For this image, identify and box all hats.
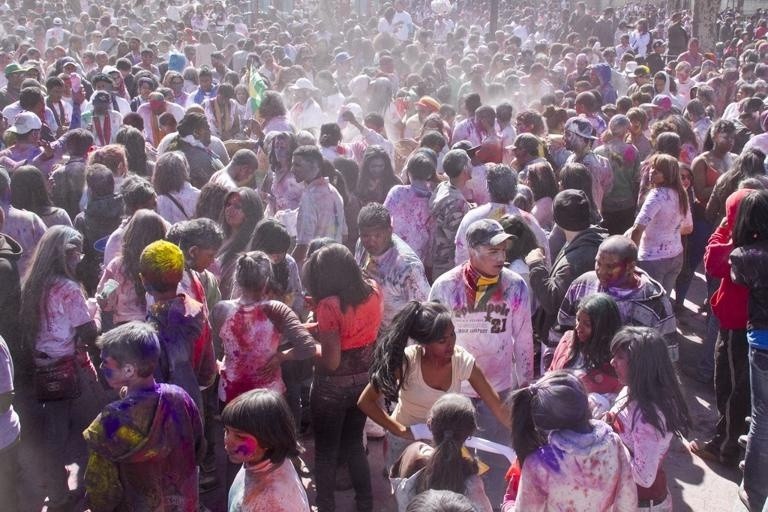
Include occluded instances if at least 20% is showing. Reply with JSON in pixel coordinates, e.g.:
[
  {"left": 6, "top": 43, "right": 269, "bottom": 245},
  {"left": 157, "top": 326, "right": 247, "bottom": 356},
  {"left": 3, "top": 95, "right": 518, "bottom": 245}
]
[
  {"left": 52, "top": 18, "right": 62, "bottom": 25},
  {"left": 336, "top": 52, "right": 353, "bottom": 64},
  {"left": 553, "top": 189, "right": 590, "bottom": 230},
  {"left": 505, "top": 134, "right": 542, "bottom": 150},
  {"left": 7, "top": 111, "right": 41, "bottom": 135},
  {"left": 628, "top": 65, "right": 650, "bottom": 79},
  {"left": 290, "top": 78, "right": 317, "bottom": 91},
  {"left": 466, "top": 219, "right": 517, "bottom": 246},
  {"left": 563, "top": 118, "right": 598, "bottom": 139},
  {"left": 414, "top": 96, "right": 439, "bottom": 113},
  {"left": 5, "top": 63, "right": 27, "bottom": 76},
  {"left": 451, "top": 140, "right": 481, "bottom": 152}
]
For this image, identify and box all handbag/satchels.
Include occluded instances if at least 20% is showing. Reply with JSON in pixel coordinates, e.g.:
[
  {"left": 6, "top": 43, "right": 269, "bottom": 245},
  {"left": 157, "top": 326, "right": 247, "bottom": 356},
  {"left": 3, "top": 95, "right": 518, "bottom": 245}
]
[{"left": 35, "top": 362, "right": 79, "bottom": 400}]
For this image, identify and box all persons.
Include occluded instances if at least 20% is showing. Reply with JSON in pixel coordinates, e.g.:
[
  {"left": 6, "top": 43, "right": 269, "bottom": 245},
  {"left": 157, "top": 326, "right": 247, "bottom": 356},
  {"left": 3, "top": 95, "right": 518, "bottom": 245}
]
[{"left": 0, "top": 0, "right": 768, "bottom": 512}]
